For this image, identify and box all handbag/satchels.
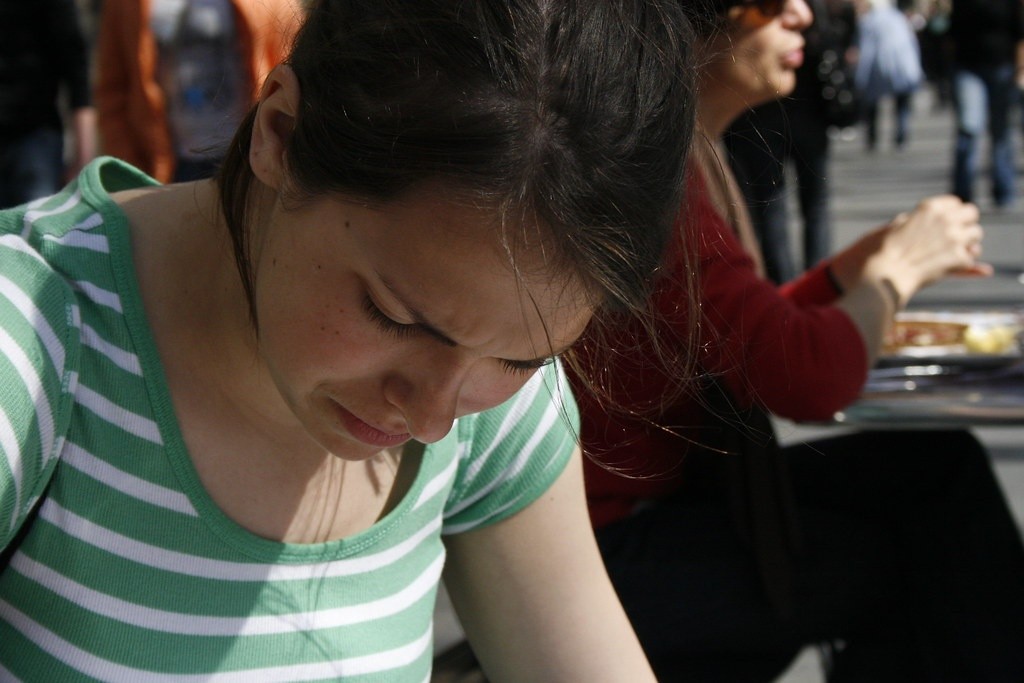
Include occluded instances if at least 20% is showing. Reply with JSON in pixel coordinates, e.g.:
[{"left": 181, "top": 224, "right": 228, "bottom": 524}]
[{"left": 817, "top": 54, "right": 859, "bottom": 128}]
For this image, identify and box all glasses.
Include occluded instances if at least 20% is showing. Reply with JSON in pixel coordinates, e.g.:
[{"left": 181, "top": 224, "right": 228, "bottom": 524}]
[{"left": 702, "top": 0, "right": 786, "bottom": 48}]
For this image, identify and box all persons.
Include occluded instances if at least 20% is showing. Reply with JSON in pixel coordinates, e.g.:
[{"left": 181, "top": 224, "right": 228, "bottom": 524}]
[{"left": 0, "top": 0, "right": 1024, "bottom": 683}]
